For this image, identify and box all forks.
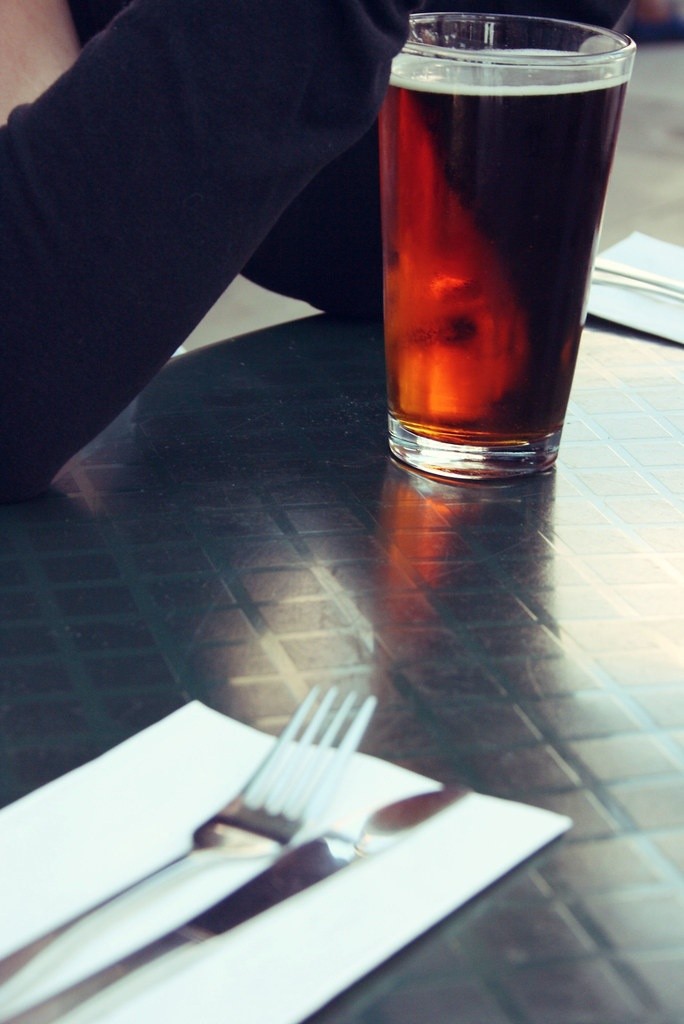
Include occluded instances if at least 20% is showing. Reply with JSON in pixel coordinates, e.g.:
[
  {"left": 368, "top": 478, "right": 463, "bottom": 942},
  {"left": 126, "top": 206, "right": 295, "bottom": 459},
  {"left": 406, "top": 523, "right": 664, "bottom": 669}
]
[{"left": 0, "top": 683, "right": 377, "bottom": 1022}]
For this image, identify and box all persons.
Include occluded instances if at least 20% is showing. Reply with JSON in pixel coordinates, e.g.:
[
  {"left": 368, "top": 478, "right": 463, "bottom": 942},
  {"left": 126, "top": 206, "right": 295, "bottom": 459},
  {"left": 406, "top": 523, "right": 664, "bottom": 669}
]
[{"left": 1, "top": 0, "right": 631, "bottom": 498}]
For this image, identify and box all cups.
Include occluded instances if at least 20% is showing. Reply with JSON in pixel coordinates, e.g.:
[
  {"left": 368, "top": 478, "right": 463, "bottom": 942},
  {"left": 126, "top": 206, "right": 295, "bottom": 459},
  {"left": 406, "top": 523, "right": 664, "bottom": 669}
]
[{"left": 375, "top": 10, "right": 636, "bottom": 490}]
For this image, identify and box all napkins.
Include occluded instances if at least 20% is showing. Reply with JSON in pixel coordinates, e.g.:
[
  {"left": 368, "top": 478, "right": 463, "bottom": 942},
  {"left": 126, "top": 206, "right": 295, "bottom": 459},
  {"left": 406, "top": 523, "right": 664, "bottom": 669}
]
[
  {"left": 588, "top": 231, "right": 684, "bottom": 348},
  {"left": 0, "top": 700, "right": 572, "bottom": 1024}
]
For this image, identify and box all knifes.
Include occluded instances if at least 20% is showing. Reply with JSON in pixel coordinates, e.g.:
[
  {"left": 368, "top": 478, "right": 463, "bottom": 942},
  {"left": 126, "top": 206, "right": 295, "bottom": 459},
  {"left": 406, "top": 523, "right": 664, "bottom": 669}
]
[{"left": 15, "top": 785, "right": 471, "bottom": 1023}]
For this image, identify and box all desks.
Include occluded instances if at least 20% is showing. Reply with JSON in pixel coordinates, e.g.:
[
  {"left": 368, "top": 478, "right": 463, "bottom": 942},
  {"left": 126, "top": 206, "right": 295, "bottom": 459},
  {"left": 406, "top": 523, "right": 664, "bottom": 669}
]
[{"left": 0, "top": 292, "right": 684, "bottom": 1023}]
